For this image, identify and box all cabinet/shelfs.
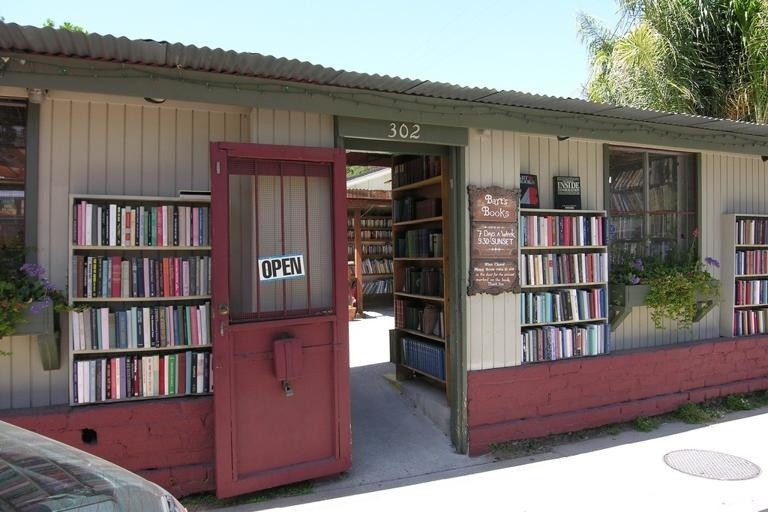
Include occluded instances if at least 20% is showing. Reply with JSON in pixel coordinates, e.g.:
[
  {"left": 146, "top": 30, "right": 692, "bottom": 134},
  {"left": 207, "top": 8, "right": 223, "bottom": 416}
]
[
  {"left": 718, "top": 210, "right": 767, "bottom": 340},
  {"left": 612, "top": 155, "right": 698, "bottom": 271},
  {"left": 345, "top": 197, "right": 393, "bottom": 312},
  {"left": 385, "top": 152, "right": 451, "bottom": 403},
  {"left": 63, "top": 190, "right": 222, "bottom": 408},
  {"left": 517, "top": 204, "right": 614, "bottom": 365}
]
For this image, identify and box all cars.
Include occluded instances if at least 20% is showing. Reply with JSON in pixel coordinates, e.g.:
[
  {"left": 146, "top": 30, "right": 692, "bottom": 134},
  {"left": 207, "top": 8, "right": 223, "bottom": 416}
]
[{"left": 0, "top": 419, "right": 190, "bottom": 510}]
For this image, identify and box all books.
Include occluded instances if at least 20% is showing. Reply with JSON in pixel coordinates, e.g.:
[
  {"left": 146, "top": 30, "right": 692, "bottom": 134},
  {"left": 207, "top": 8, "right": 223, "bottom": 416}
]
[
  {"left": 394, "top": 153, "right": 445, "bottom": 380},
  {"left": 72, "top": 200, "right": 213, "bottom": 405},
  {"left": 734, "top": 218, "right": 768, "bottom": 337},
  {"left": 345, "top": 214, "right": 393, "bottom": 296},
  {"left": 610, "top": 154, "right": 677, "bottom": 272},
  {"left": 518, "top": 215, "right": 608, "bottom": 363}
]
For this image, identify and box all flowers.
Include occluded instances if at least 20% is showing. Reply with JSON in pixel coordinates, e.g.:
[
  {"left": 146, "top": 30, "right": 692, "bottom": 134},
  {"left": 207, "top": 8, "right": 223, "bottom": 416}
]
[
  {"left": 0, "top": 254, "right": 94, "bottom": 358},
  {"left": 614, "top": 226, "right": 722, "bottom": 290}
]
